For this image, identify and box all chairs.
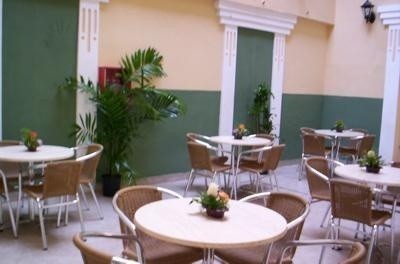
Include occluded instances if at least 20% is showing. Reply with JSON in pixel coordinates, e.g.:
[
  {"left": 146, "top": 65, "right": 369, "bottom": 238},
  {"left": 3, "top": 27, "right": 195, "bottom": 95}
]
[
  {"left": 296, "top": 125, "right": 376, "bottom": 179},
  {"left": 210, "top": 192, "right": 310, "bottom": 264},
  {"left": 304, "top": 156, "right": 345, "bottom": 227},
  {"left": 375, "top": 163, "right": 400, "bottom": 219},
  {"left": 0, "top": 140, "right": 104, "bottom": 249},
  {"left": 328, "top": 178, "right": 392, "bottom": 264},
  {"left": 112, "top": 185, "right": 204, "bottom": 264},
  {"left": 278, "top": 239, "right": 367, "bottom": 264},
  {"left": 187, "top": 132, "right": 286, "bottom": 196},
  {"left": 73, "top": 231, "right": 146, "bottom": 264}
]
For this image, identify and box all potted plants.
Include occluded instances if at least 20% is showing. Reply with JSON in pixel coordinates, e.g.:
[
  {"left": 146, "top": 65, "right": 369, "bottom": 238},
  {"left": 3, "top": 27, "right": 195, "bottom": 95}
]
[
  {"left": 54, "top": 47, "right": 186, "bottom": 197},
  {"left": 245, "top": 80, "right": 281, "bottom": 175},
  {"left": 334, "top": 121, "right": 344, "bottom": 132}
]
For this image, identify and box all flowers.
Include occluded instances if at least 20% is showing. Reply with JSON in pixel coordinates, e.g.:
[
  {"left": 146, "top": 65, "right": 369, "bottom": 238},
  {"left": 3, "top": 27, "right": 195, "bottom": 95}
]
[
  {"left": 232, "top": 124, "right": 247, "bottom": 139},
  {"left": 20, "top": 128, "right": 40, "bottom": 149},
  {"left": 190, "top": 181, "right": 230, "bottom": 208},
  {"left": 358, "top": 149, "right": 382, "bottom": 167}
]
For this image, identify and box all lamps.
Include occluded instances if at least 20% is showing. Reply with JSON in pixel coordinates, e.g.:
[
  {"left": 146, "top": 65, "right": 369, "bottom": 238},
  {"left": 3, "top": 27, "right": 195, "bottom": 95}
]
[{"left": 360, "top": 0, "right": 378, "bottom": 25}]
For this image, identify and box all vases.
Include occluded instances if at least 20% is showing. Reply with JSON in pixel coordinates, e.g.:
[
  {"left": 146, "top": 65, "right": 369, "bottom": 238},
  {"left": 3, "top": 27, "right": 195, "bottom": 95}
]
[
  {"left": 207, "top": 207, "right": 223, "bottom": 218},
  {"left": 365, "top": 164, "right": 380, "bottom": 174}
]
